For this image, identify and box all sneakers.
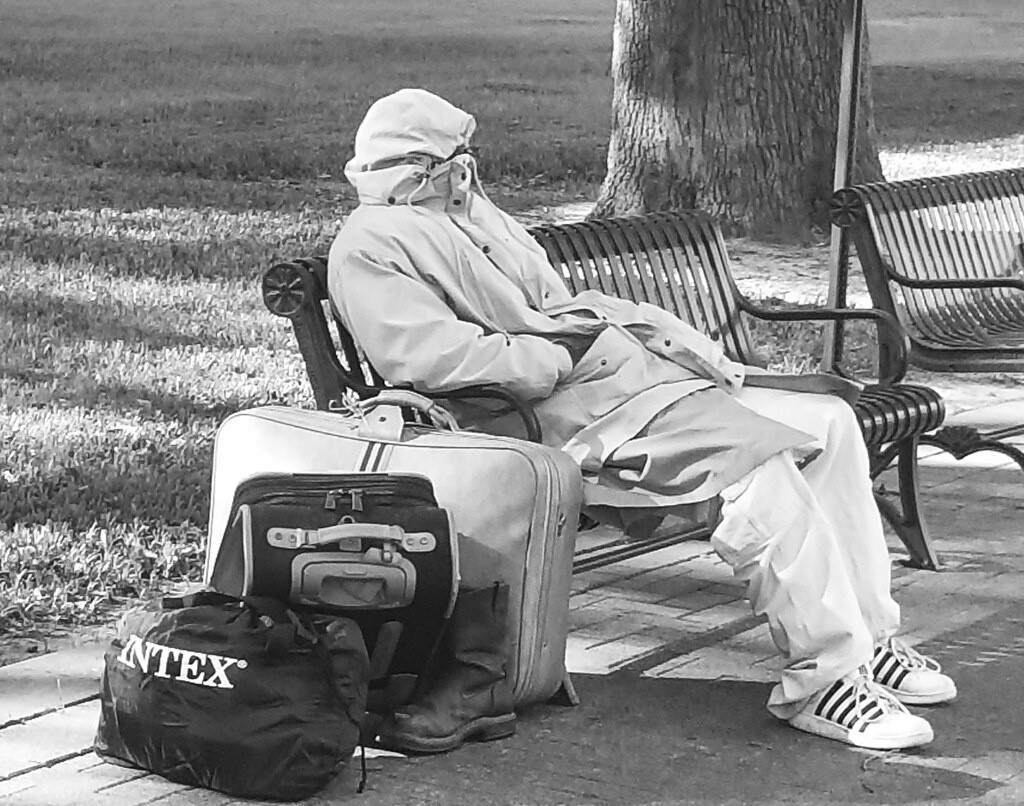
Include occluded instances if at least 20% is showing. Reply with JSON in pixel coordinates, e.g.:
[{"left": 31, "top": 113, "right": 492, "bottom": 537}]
[
  {"left": 869, "top": 636, "right": 958, "bottom": 703},
  {"left": 787, "top": 661, "right": 935, "bottom": 750}
]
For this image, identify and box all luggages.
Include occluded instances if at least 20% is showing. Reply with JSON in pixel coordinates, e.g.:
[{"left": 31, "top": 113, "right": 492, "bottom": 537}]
[{"left": 205, "top": 472, "right": 460, "bottom": 733}]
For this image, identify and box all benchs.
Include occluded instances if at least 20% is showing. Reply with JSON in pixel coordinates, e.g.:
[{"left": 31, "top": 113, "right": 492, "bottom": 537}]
[
  {"left": 262, "top": 209, "right": 945, "bottom": 706},
  {"left": 830, "top": 166, "right": 1024, "bottom": 473}
]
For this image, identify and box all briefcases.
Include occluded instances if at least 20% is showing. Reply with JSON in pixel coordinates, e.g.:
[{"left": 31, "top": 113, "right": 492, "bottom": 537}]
[{"left": 202, "top": 387, "right": 584, "bottom": 707}]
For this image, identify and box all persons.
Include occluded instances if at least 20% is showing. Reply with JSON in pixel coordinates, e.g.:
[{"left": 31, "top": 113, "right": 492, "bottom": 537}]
[{"left": 329, "top": 87, "right": 957, "bottom": 749}]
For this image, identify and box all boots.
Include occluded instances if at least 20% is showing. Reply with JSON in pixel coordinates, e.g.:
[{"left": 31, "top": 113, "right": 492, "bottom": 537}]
[{"left": 376, "top": 580, "right": 516, "bottom": 753}]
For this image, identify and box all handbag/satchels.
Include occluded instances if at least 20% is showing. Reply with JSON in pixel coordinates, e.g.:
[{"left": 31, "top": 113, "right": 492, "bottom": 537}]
[{"left": 91, "top": 587, "right": 370, "bottom": 802}]
